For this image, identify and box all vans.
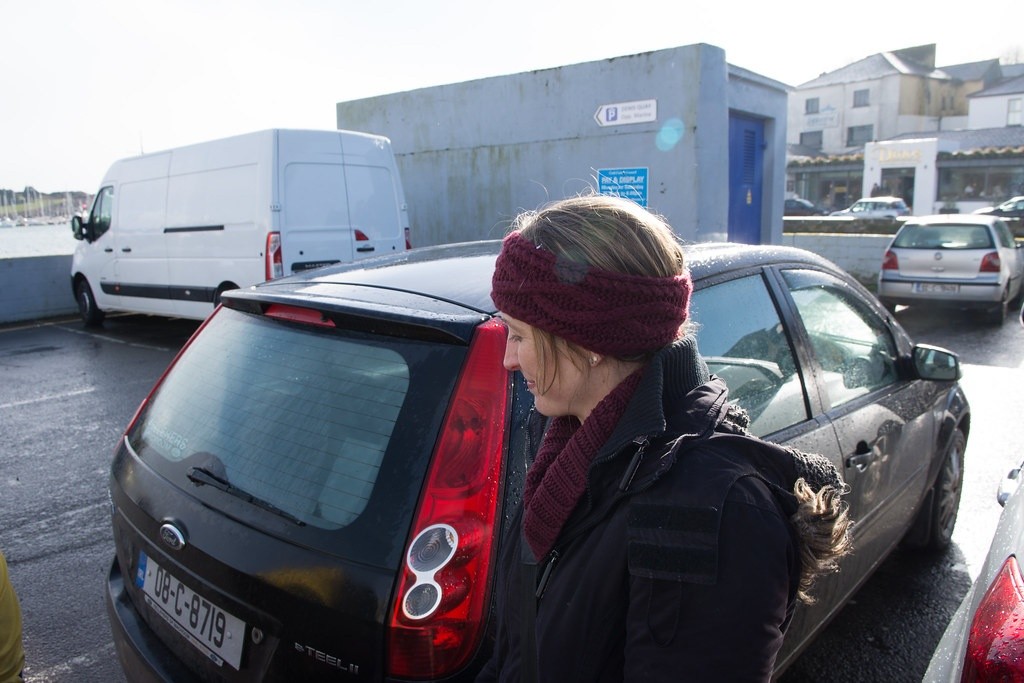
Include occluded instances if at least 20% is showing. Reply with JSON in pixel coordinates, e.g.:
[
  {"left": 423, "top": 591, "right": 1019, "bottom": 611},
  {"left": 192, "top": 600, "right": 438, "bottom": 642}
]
[{"left": 68, "top": 126, "right": 410, "bottom": 332}]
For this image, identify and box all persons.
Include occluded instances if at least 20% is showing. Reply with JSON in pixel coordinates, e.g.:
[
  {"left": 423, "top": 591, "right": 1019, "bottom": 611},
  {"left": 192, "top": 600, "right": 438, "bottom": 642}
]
[{"left": 491, "top": 192, "right": 854, "bottom": 683}]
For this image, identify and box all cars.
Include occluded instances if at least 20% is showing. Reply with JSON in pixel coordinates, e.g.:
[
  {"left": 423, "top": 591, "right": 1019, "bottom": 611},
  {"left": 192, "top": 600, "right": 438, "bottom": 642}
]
[
  {"left": 877, "top": 214, "right": 1023, "bottom": 327},
  {"left": 828, "top": 196, "right": 911, "bottom": 222},
  {"left": 784, "top": 198, "right": 830, "bottom": 217},
  {"left": 971, "top": 196, "right": 1024, "bottom": 218},
  {"left": 102, "top": 235, "right": 976, "bottom": 683}
]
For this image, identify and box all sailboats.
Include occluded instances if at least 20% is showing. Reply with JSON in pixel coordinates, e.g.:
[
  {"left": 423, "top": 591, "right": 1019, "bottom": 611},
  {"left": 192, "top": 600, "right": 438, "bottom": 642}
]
[{"left": 0, "top": 183, "right": 98, "bottom": 227}]
